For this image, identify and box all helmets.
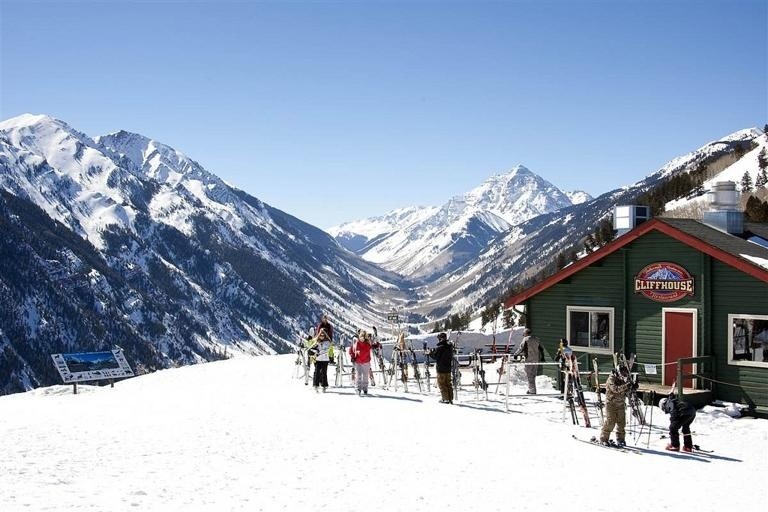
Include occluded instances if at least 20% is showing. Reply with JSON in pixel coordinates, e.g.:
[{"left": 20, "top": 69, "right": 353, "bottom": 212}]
[{"left": 658, "top": 398, "right": 668, "bottom": 411}]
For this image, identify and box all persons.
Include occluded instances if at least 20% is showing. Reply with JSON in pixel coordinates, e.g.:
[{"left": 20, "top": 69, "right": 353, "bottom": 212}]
[
  {"left": 303, "top": 314, "right": 334, "bottom": 393},
  {"left": 348, "top": 328, "right": 383, "bottom": 395},
  {"left": 513, "top": 328, "right": 545, "bottom": 395},
  {"left": 425, "top": 333, "right": 455, "bottom": 404},
  {"left": 658, "top": 397, "right": 696, "bottom": 453},
  {"left": 600, "top": 364, "right": 639, "bottom": 448},
  {"left": 553, "top": 338, "right": 576, "bottom": 400}
]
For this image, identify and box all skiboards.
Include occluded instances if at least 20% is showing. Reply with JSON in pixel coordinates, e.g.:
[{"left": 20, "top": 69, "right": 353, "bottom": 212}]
[
  {"left": 649, "top": 441, "right": 714, "bottom": 459},
  {"left": 369, "top": 325, "right": 516, "bottom": 401},
  {"left": 561, "top": 352, "right": 645, "bottom": 428},
  {"left": 334, "top": 330, "right": 361, "bottom": 386},
  {"left": 572, "top": 435, "right": 643, "bottom": 456}
]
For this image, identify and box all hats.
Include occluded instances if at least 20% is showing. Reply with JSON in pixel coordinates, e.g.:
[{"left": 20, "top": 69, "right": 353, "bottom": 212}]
[
  {"left": 522, "top": 328, "right": 531, "bottom": 336},
  {"left": 437, "top": 333, "right": 449, "bottom": 341}
]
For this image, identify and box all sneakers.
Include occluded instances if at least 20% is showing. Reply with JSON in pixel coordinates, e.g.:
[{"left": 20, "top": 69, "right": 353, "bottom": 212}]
[
  {"left": 439, "top": 400, "right": 453, "bottom": 404},
  {"left": 666, "top": 446, "right": 692, "bottom": 452},
  {"left": 599, "top": 439, "right": 626, "bottom": 446},
  {"left": 355, "top": 389, "right": 367, "bottom": 396}
]
